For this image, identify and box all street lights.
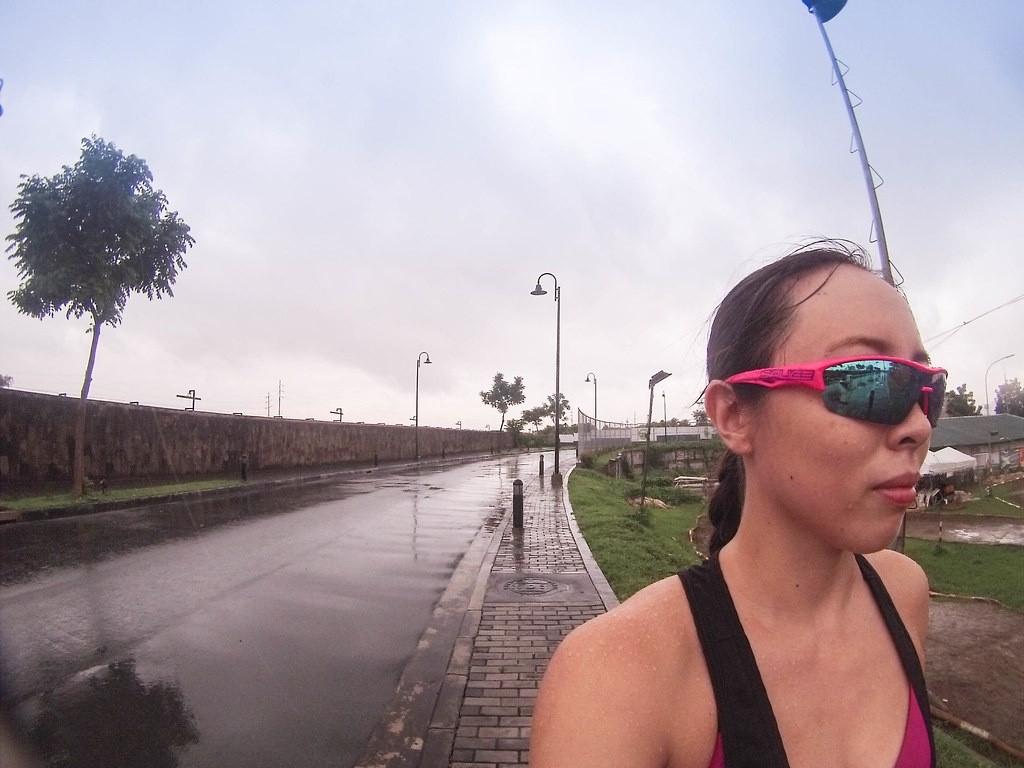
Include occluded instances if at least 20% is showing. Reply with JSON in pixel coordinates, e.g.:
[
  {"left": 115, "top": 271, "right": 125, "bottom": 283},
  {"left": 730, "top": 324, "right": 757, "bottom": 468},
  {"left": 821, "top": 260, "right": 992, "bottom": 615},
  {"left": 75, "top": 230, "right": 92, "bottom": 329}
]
[
  {"left": 985, "top": 354, "right": 1015, "bottom": 476},
  {"left": 662, "top": 391, "right": 667, "bottom": 443},
  {"left": 415, "top": 352, "right": 432, "bottom": 461},
  {"left": 585, "top": 373, "right": 598, "bottom": 451},
  {"left": 531, "top": 273, "right": 563, "bottom": 485},
  {"left": 801, "top": 0, "right": 903, "bottom": 289}
]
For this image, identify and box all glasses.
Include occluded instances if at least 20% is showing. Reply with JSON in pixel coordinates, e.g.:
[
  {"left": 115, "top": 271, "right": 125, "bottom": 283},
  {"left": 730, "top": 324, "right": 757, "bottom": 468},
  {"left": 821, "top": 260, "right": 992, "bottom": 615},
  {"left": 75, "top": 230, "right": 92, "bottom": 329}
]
[{"left": 724, "top": 355, "right": 948, "bottom": 428}]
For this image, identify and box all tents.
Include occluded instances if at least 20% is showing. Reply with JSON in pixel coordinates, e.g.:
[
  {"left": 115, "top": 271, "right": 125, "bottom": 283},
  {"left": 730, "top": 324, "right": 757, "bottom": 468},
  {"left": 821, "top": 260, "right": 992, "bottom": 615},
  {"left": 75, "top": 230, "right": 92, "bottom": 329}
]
[{"left": 919, "top": 446, "right": 977, "bottom": 490}]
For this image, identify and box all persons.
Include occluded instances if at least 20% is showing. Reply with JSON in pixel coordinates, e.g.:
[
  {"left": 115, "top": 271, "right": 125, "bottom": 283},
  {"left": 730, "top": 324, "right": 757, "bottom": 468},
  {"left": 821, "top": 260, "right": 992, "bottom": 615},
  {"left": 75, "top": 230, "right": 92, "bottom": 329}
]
[{"left": 527, "top": 248, "right": 940, "bottom": 768}]
